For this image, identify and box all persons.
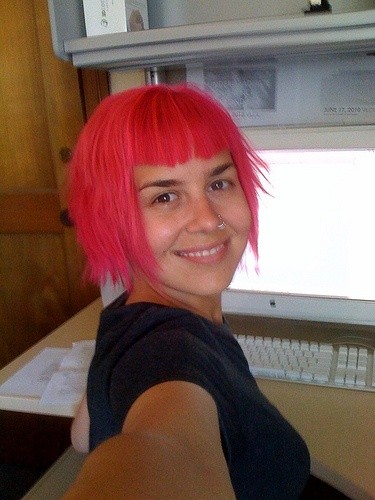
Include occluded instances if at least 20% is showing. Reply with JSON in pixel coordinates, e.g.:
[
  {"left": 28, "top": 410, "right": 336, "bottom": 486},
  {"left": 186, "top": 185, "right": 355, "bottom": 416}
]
[{"left": 57, "top": 82, "right": 313, "bottom": 500}]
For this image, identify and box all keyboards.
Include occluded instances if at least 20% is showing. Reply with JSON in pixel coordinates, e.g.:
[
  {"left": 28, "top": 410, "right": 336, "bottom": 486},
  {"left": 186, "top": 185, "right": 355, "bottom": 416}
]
[{"left": 232, "top": 333, "right": 375, "bottom": 393}]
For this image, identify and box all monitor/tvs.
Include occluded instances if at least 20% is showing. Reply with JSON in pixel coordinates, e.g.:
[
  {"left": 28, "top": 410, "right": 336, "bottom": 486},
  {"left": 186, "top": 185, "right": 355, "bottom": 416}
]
[{"left": 220, "top": 123, "right": 375, "bottom": 325}]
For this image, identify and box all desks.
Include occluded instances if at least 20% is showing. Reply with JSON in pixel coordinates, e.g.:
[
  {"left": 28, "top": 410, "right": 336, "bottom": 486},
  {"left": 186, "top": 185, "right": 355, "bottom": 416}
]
[{"left": 3, "top": 285, "right": 375, "bottom": 500}]
